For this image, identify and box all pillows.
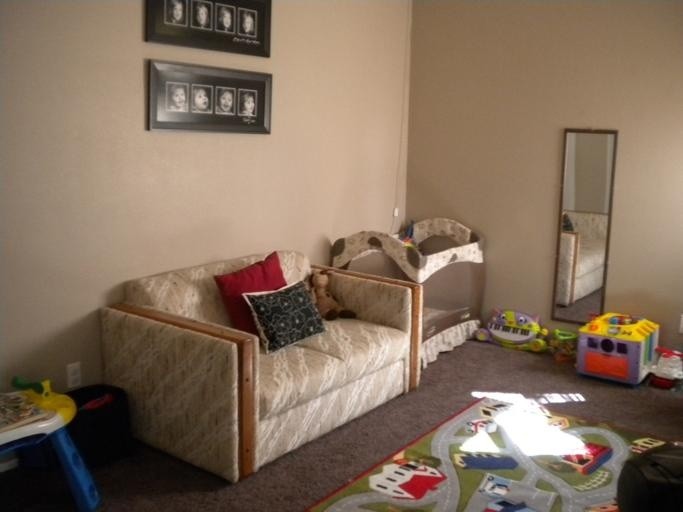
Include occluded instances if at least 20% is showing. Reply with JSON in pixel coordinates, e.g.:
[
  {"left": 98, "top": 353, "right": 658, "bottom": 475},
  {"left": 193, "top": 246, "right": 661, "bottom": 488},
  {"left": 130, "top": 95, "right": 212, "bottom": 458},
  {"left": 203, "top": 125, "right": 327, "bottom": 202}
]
[{"left": 213, "top": 252, "right": 326, "bottom": 355}]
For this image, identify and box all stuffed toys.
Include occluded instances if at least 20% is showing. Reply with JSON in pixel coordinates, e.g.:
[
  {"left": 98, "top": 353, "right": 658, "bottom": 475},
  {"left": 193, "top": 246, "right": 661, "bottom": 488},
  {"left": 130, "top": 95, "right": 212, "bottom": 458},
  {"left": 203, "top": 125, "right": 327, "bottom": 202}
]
[{"left": 306, "top": 270, "right": 359, "bottom": 321}]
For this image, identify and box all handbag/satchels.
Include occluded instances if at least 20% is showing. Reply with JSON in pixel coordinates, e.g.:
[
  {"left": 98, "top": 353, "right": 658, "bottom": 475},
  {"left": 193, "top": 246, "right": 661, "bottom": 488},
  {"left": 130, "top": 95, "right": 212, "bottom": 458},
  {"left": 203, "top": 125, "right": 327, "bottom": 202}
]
[{"left": 615, "top": 444, "right": 682, "bottom": 512}]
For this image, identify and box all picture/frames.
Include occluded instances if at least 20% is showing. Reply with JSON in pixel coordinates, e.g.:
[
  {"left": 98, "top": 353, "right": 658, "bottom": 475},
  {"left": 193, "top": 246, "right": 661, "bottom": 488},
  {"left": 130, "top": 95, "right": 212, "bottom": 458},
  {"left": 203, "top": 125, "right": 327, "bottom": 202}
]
[
  {"left": 148, "top": 61, "right": 273, "bottom": 134},
  {"left": 145, "top": 0, "right": 272, "bottom": 58}
]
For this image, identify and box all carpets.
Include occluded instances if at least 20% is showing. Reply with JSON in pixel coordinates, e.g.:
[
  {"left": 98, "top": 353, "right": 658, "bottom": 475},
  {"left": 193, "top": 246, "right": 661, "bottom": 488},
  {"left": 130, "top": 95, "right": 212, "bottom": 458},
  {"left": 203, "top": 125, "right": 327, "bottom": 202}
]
[{"left": 301, "top": 397, "right": 683, "bottom": 510}]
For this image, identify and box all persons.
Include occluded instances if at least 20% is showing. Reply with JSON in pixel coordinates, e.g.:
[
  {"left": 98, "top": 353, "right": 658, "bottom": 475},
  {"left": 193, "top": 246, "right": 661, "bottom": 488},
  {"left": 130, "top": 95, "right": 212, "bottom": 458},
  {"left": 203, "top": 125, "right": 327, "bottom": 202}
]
[
  {"left": 241, "top": 92, "right": 254, "bottom": 114},
  {"left": 193, "top": 88, "right": 212, "bottom": 113},
  {"left": 241, "top": 11, "right": 255, "bottom": 34},
  {"left": 195, "top": 2, "right": 210, "bottom": 27},
  {"left": 166, "top": 0, "right": 185, "bottom": 24},
  {"left": 218, "top": 7, "right": 232, "bottom": 31},
  {"left": 167, "top": 84, "right": 186, "bottom": 111},
  {"left": 217, "top": 90, "right": 233, "bottom": 113}
]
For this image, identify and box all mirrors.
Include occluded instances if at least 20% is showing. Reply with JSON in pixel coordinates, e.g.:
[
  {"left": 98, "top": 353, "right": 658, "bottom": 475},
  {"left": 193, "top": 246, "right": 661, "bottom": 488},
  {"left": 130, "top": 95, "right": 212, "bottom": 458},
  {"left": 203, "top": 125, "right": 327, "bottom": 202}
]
[{"left": 551, "top": 129, "right": 619, "bottom": 325}]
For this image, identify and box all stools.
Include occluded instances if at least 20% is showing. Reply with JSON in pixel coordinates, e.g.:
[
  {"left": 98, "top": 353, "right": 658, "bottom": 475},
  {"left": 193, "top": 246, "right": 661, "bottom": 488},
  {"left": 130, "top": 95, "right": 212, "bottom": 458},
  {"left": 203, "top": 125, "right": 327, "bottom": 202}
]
[{"left": 1, "top": 378, "right": 103, "bottom": 511}]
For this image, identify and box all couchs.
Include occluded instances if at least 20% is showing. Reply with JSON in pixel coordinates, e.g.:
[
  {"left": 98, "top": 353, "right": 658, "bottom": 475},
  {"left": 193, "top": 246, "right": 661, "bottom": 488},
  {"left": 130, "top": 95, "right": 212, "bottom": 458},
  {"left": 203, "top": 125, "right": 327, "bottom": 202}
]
[
  {"left": 554, "top": 208, "right": 608, "bottom": 307},
  {"left": 97, "top": 254, "right": 424, "bottom": 486}
]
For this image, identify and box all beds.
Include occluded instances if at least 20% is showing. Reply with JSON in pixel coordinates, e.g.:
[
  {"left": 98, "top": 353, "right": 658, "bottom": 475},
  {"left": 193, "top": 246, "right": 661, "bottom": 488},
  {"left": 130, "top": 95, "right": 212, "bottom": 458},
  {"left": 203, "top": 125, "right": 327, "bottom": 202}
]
[{"left": 330, "top": 218, "right": 487, "bottom": 367}]
